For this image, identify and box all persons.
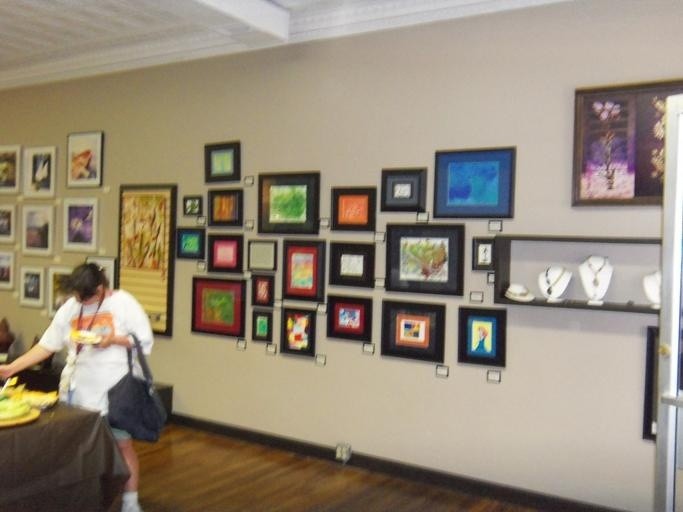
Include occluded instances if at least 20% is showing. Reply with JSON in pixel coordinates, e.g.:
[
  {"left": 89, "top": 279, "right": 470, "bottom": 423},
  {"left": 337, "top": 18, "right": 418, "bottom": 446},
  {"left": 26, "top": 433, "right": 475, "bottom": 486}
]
[{"left": 0, "top": 261, "right": 154, "bottom": 511}]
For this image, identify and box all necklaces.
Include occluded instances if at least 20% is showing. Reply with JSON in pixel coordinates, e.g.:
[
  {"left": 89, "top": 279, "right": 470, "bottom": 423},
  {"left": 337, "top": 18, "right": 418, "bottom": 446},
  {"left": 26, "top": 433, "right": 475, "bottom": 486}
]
[
  {"left": 541, "top": 265, "right": 567, "bottom": 296},
  {"left": 508, "top": 283, "right": 529, "bottom": 298},
  {"left": 586, "top": 254, "right": 608, "bottom": 288}
]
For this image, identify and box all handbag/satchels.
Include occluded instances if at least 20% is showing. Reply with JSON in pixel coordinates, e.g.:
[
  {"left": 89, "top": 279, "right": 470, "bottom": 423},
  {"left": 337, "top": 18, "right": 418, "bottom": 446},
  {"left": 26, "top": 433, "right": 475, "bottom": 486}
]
[{"left": 109, "top": 372, "right": 167, "bottom": 441}]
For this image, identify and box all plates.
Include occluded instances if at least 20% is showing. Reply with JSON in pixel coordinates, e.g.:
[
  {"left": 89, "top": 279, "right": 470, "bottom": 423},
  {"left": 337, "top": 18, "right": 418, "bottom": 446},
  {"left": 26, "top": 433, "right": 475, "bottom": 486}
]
[
  {"left": 67, "top": 329, "right": 102, "bottom": 346},
  {"left": 0, "top": 408, "right": 40, "bottom": 429}
]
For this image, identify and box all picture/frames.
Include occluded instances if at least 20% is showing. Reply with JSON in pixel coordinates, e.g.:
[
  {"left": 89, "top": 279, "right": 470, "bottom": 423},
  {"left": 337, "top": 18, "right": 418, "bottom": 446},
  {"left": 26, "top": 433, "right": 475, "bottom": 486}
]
[
  {"left": 568, "top": 78, "right": 683, "bottom": 211},
  {"left": 640, "top": 324, "right": 661, "bottom": 438}
]
